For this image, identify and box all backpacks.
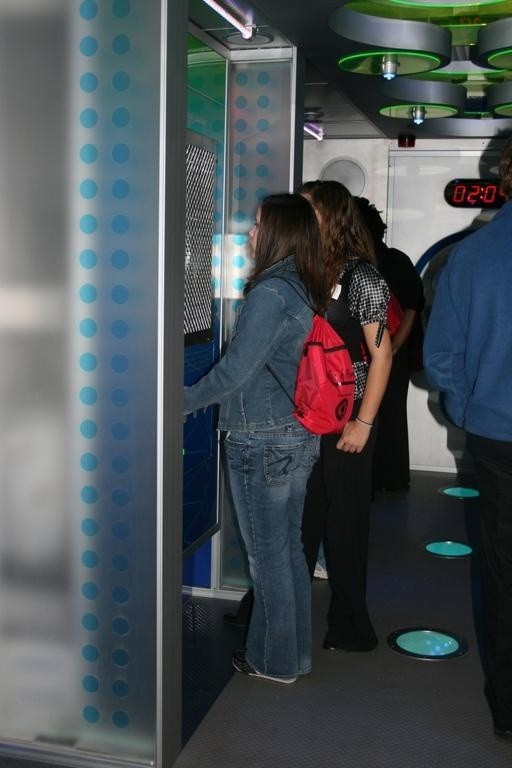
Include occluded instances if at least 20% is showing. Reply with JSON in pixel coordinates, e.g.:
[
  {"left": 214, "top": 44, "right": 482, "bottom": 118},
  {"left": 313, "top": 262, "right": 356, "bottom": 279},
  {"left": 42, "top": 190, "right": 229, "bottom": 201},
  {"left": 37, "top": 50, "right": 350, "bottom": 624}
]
[{"left": 264, "top": 276, "right": 355, "bottom": 436}]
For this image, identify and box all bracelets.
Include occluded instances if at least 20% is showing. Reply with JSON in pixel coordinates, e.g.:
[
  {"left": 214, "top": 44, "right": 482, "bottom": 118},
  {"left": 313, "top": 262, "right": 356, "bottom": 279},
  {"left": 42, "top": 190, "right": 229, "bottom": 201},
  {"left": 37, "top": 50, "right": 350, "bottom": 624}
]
[{"left": 356, "top": 416, "right": 374, "bottom": 432}]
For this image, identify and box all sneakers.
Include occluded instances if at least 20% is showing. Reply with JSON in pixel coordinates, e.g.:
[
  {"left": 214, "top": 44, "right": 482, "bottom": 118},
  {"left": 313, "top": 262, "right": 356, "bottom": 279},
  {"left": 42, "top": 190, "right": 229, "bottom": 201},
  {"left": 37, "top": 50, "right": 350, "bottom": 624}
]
[
  {"left": 322, "top": 632, "right": 338, "bottom": 651},
  {"left": 232, "top": 649, "right": 297, "bottom": 683},
  {"left": 224, "top": 613, "right": 249, "bottom": 629}
]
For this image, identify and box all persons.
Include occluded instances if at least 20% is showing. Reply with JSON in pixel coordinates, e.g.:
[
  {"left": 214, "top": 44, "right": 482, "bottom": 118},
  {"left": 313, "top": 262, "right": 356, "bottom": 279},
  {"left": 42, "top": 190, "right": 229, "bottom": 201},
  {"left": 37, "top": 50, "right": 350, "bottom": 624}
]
[
  {"left": 186, "top": 193, "right": 329, "bottom": 683},
  {"left": 222, "top": 181, "right": 393, "bottom": 650},
  {"left": 351, "top": 195, "right": 423, "bottom": 492},
  {"left": 423, "top": 144, "right": 512, "bottom": 740}
]
